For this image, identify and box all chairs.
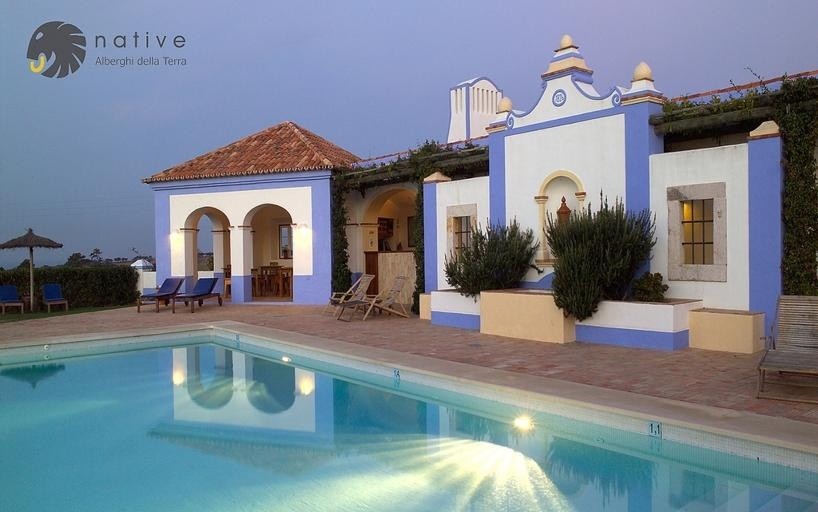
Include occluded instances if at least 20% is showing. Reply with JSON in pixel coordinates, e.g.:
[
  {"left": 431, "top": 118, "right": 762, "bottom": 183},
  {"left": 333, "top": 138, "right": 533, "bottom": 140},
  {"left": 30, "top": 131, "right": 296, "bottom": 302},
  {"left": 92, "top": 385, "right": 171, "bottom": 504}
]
[
  {"left": 321, "top": 272, "right": 411, "bottom": 324},
  {"left": 755, "top": 295, "right": 817, "bottom": 407},
  {"left": 136, "top": 276, "right": 223, "bottom": 315},
  {"left": 1, "top": 283, "right": 69, "bottom": 316},
  {"left": 220, "top": 260, "right": 293, "bottom": 299}
]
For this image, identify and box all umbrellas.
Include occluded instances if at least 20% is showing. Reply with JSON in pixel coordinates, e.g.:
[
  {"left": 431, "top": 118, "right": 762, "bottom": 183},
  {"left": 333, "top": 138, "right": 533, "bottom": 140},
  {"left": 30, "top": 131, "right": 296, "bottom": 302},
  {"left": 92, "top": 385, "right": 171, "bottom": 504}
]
[{"left": 0, "top": 229, "right": 63, "bottom": 313}]
